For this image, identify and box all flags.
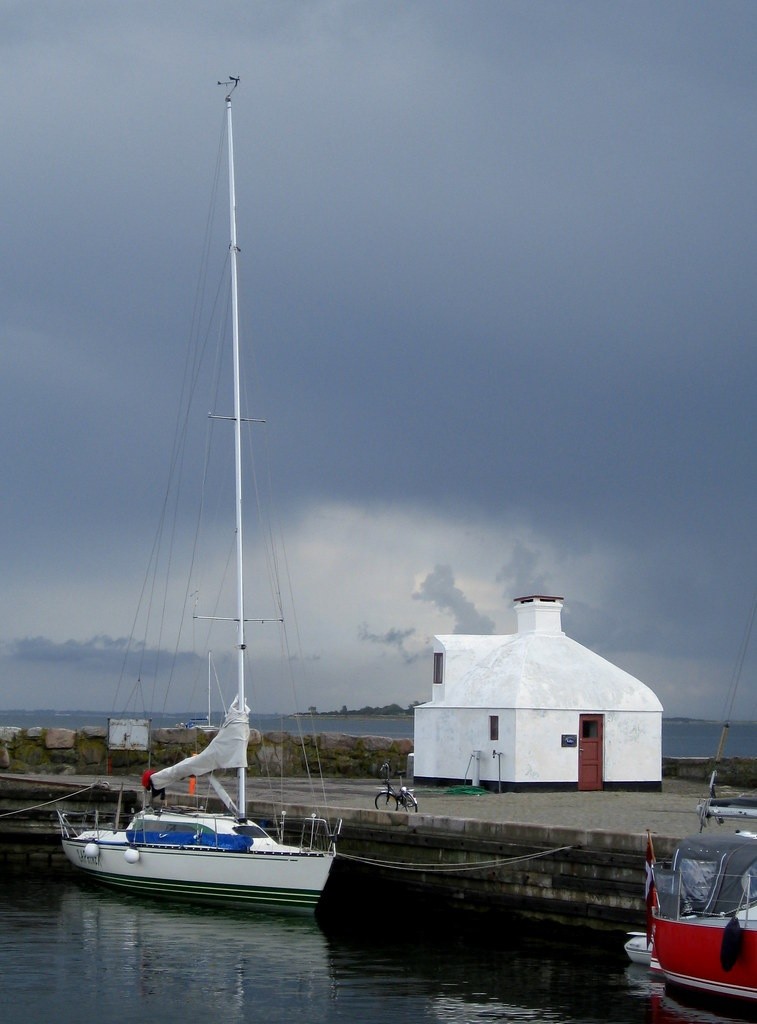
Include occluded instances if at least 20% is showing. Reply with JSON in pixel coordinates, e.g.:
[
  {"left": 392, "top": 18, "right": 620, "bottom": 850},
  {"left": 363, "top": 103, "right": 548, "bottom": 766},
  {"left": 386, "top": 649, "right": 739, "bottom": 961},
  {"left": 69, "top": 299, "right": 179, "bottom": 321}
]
[{"left": 644, "top": 835, "right": 659, "bottom": 948}]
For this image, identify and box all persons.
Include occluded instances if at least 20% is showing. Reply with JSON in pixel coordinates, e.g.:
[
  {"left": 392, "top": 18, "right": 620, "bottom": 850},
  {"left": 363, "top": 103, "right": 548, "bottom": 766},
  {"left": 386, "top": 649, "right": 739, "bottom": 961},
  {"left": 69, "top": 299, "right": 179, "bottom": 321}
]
[{"left": 176, "top": 721, "right": 188, "bottom": 728}]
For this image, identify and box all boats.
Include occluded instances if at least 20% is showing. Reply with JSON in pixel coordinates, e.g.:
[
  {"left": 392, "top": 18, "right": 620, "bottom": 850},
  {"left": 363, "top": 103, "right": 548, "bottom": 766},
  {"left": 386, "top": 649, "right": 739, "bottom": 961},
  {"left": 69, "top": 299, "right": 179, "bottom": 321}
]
[
  {"left": 639, "top": 604, "right": 757, "bottom": 1007},
  {"left": 623, "top": 931, "right": 655, "bottom": 966}
]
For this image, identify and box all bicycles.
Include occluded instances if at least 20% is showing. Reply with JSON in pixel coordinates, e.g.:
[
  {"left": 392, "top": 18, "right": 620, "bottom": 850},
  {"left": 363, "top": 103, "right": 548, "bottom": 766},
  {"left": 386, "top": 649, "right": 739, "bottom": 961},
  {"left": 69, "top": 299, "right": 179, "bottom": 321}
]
[{"left": 375, "top": 758, "right": 418, "bottom": 813}]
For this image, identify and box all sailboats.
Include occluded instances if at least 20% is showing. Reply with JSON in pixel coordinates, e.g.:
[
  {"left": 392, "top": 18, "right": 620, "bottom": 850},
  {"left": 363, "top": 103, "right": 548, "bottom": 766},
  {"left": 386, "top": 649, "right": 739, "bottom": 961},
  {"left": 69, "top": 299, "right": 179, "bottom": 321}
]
[
  {"left": 55, "top": 67, "right": 343, "bottom": 900},
  {"left": 177, "top": 649, "right": 228, "bottom": 732}
]
